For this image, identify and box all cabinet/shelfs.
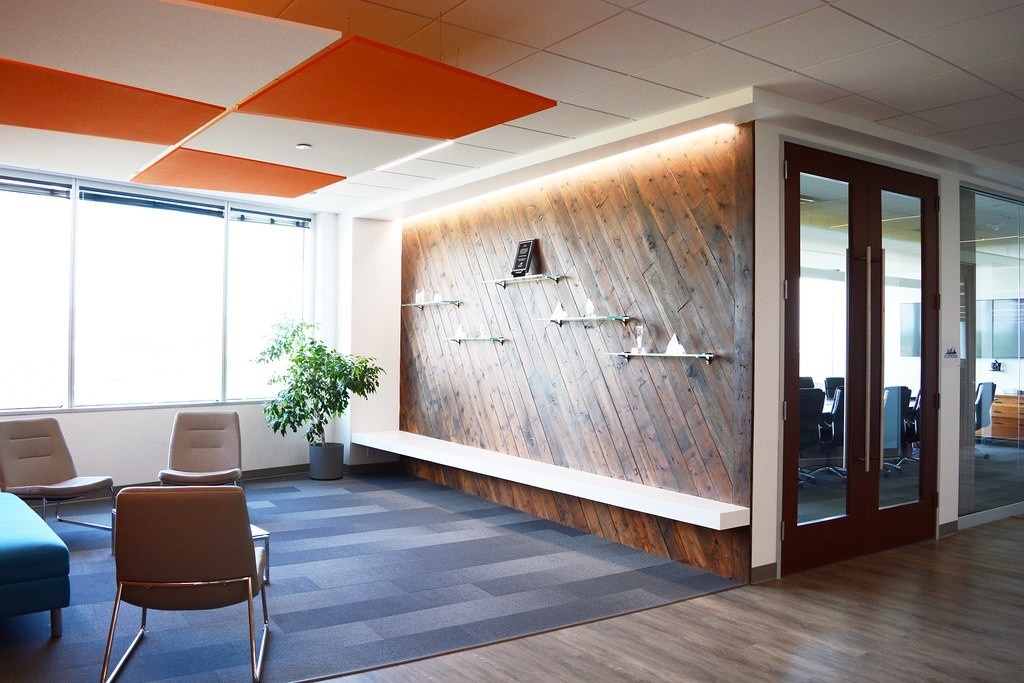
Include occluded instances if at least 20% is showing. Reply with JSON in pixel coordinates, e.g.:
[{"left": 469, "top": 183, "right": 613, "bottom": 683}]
[{"left": 975, "top": 393, "right": 1024, "bottom": 445}]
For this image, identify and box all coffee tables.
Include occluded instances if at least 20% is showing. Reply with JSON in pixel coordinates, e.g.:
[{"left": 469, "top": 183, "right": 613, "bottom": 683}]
[{"left": 111, "top": 509, "right": 269, "bottom": 585}]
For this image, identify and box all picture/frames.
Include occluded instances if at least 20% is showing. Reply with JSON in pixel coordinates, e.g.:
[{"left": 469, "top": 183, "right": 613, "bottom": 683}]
[{"left": 512, "top": 239, "right": 537, "bottom": 273}]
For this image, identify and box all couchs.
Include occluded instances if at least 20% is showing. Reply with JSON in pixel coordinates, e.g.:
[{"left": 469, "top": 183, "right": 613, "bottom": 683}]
[{"left": 0, "top": 493, "right": 72, "bottom": 638}]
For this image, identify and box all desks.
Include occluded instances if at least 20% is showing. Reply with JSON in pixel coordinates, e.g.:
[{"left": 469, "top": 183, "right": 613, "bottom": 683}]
[{"left": 799, "top": 395, "right": 918, "bottom": 469}]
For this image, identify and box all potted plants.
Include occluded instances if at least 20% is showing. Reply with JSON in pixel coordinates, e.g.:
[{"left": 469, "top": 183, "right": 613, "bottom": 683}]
[{"left": 251, "top": 315, "right": 385, "bottom": 480}]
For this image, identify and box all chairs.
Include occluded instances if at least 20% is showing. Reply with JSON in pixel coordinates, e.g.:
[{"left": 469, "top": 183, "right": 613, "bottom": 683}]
[
  {"left": 895, "top": 389, "right": 921, "bottom": 468},
  {"left": 882, "top": 386, "right": 911, "bottom": 476},
  {"left": 799, "top": 388, "right": 826, "bottom": 488},
  {"left": 799, "top": 376, "right": 814, "bottom": 389},
  {"left": 825, "top": 377, "right": 844, "bottom": 398},
  {"left": 975, "top": 381, "right": 997, "bottom": 459},
  {"left": 100, "top": 486, "right": 269, "bottom": 683},
  {"left": 0, "top": 415, "right": 116, "bottom": 530},
  {"left": 158, "top": 409, "right": 243, "bottom": 487},
  {"left": 807, "top": 386, "right": 846, "bottom": 482}
]
